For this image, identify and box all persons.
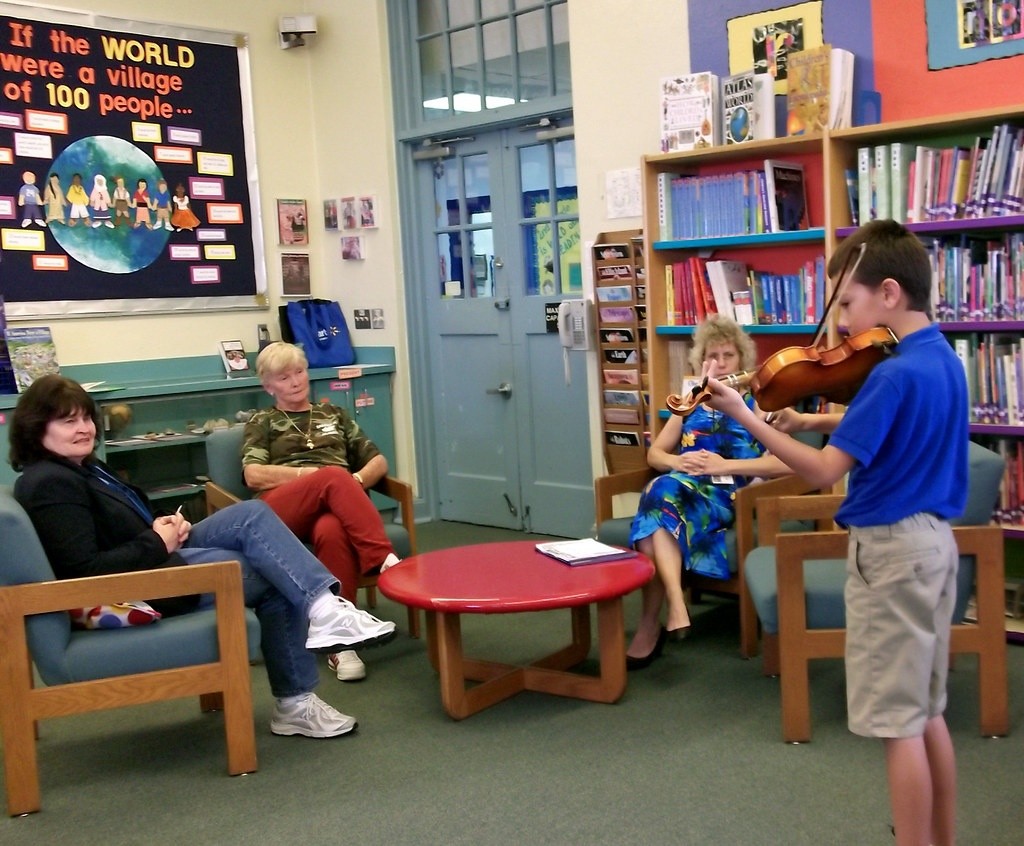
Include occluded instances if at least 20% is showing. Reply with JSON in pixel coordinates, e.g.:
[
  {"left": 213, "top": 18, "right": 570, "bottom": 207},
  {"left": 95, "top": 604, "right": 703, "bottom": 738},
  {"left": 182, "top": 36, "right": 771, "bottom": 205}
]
[
  {"left": 624, "top": 315, "right": 797, "bottom": 673},
  {"left": 702, "top": 216, "right": 971, "bottom": 846},
  {"left": 243, "top": 342, "right": 403, "bottom": 681},
  {"left": 8, "top": 375, "right": 397, "bottom": 738}
]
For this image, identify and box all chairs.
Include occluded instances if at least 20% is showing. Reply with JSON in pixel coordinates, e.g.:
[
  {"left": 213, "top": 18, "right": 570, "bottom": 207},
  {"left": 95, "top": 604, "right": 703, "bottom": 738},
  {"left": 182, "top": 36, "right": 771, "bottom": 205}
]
[
  {"left": 758, "top": 437, "right": 1006, "bottom": 743},
  {"left": 594, "top": 408, "right": 846, "bottom": 662},
  {"left": 1, "top": 483, "right": 258, "bottom": 815},
  {"left": 205, "top": 422, "right": 420, "bottom": 665}
]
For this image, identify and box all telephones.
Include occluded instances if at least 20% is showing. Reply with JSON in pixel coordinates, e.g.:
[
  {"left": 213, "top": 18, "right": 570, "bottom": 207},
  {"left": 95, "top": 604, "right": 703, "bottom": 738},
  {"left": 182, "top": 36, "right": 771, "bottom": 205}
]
[{"left": 558, "top": 299, "right": 595, "bottom": 351}]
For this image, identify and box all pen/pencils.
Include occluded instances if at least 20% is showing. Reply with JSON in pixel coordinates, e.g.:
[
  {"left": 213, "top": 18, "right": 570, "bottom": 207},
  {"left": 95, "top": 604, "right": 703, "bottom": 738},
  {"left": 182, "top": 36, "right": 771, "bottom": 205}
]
[{"left": 177, "top": 505, "right": 183, "bottom": 513}]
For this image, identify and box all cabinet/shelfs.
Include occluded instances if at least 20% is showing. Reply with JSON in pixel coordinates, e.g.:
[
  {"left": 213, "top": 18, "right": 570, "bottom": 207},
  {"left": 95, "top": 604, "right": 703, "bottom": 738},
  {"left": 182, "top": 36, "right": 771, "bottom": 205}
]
[
  {"left": 642, "top": 106, "right": 1024, "bottom": 648},
  {"left": 592, "top": 227, "right": 646, "bottom": 475},
  {"left": 0, "top": 364, "right": 399, "bottom": 528}
]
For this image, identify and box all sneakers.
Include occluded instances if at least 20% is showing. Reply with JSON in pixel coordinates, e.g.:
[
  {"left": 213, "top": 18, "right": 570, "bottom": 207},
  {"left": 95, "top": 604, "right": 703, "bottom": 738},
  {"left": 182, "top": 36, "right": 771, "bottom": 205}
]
[
  {"left": 305, "top": 596, "right": 396, "bottom": 653},
  {"left": 324, "top": 648, "right": 366, "bottom": 681},
  {"left": 380, "top": 552, "right": 401, "bottom": 574},
  {"left": 271, "top": 692, "right": 358, "bottom": 738}
]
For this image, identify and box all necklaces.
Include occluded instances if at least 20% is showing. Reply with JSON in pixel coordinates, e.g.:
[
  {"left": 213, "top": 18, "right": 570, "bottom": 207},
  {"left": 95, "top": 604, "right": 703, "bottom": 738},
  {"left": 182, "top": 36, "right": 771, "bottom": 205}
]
[{"left": 280, "top": 404, "right": 315, "bottom": 449}]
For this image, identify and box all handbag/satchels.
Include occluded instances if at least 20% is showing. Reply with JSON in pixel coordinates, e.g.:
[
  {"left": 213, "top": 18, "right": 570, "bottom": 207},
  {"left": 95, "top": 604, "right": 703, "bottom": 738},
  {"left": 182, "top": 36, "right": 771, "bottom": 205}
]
[{"left": 279, "top": 300, "right": 357, "bottom": 367}]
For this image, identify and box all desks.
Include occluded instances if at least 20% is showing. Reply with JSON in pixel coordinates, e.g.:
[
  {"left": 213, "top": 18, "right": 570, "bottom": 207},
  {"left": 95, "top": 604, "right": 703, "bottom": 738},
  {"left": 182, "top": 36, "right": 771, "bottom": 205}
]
[{"left": 377, "top": 540, "right": 656, "bottom": 719}]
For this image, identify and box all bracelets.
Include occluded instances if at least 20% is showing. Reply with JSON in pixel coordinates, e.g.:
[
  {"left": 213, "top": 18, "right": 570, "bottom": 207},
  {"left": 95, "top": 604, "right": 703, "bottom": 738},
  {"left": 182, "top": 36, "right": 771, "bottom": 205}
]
[
  {"left": 353, "top": 472, "right": 364, "bottom": 487},
  {"left": 295, "top": 467, "right": 303, "bottom": 479}
]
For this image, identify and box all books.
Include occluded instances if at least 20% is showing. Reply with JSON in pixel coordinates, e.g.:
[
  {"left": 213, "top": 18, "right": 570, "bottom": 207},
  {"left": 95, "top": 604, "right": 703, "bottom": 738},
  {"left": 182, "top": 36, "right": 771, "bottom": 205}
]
[
  {"left": 844, "top": 122, "right": 1024, "bottom": 226},
  {"left": 592, "top": 234, "right": 651, "bottom": 448},
  {"left": 671, "top": 333, "right": 1024, "bottom": 427},
  {"left": 659, "top": 44, "right": 854, "bottom": 155},
  {"left": 978, "top": 439, "right": 1024, "bottom": 529},
  {"left": 658, "top": 160, "right": 810, "bottom": 242},
  {"left": 3, "top": 326, "right": 62, "bottom": 395},
  {"left": 219, "top": 339, "right": 250, "bottom": 372},
  {"left": 663, "top": 232, "right": 1024, "bottom": 325}
]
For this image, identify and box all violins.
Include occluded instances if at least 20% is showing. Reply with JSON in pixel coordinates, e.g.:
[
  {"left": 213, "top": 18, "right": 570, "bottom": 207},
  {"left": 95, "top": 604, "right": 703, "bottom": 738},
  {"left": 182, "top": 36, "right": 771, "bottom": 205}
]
[{"left": 664, "top": 323, "right": 900, "bottom": 419}]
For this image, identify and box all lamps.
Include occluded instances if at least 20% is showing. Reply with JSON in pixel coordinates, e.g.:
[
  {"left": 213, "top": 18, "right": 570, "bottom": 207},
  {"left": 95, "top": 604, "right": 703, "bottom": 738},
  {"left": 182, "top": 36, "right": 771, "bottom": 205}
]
[{"left": 278, "top": 15, "right": 317, "bottom": 49}]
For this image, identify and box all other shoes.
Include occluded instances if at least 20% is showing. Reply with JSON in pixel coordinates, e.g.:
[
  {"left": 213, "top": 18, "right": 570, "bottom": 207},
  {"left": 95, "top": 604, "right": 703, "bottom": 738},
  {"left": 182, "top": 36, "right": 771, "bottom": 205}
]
[
  {"left": 666, "top": 604, "right": 693, "bottom": 643},
  {"left": 625, "top": 624, "right": 665, "bottom": 670}
]
